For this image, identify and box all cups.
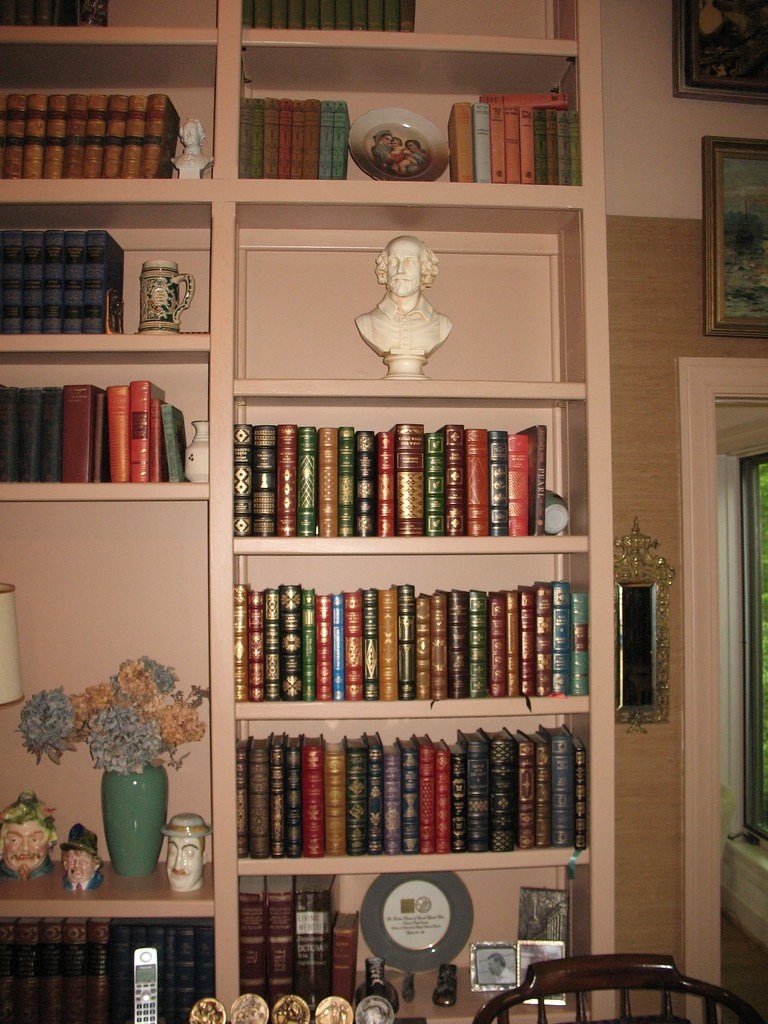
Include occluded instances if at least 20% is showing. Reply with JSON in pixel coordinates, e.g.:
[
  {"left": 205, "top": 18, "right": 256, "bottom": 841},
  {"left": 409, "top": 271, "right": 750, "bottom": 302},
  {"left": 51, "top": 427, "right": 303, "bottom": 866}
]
[{"left": 137, "top": 259, "right": 195, "bottom": 336}]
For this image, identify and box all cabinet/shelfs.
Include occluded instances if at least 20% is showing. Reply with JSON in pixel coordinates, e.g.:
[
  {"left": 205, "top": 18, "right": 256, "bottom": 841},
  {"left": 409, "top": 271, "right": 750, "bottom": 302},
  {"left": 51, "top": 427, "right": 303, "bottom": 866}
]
[{"left": 0, "top": 0, "right": 618, "bottom": 1024}]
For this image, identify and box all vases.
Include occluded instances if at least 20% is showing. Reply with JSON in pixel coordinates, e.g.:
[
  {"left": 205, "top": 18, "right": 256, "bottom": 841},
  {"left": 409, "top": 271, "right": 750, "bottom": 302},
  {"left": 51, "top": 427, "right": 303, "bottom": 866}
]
[{"left": 101, "top": 765, "right": 170, "bottom": 879}]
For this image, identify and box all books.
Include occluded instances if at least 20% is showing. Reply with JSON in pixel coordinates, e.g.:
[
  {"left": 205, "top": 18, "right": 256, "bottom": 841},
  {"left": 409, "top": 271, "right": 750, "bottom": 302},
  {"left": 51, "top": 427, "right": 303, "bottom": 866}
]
[
  {"left": 236, "top": 724, "right": 587, "bottom": 860},
  {"left": 448, "top": 94, "right": 582, "bottom": 185},
  {"left": 0, "top": 93, "right": 180, "bottom": 179},
  {"left": 0, "top": 0, "right": 108, "bottom": 27},
  {"left": 0, "top": 385, "right": 186, "bottom": 483},
  {"left": 234, "top": 422, "right": 547, "bottom": 536},
  {"left": 242, "top": 0, "right": 415, "bottom": 32},
  {"left": 238, "top": 874, "right": 359, "bottom": 1019},
  {"left": 0, "top": 230, "right": 123, "bottom": 332},
  {"left": 234, "top": 582, "right": 588, "bottom": 702},
  {"left": 0, "top": 916, "right": 216, "bottom": 1024},
  {"left": 238, "top": 97, "right": 349, "bottom": 179}
]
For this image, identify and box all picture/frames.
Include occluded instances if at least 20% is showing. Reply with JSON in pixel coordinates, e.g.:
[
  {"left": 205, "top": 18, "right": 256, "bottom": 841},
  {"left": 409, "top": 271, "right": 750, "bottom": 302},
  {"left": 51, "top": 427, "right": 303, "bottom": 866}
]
[
  {"left": 518, "top": 941, "right": 567, "bottom": 1005},
  {"left": 468, "top": 940, "right": 518, "bottom": 992},
  {"left": 671, "top": 0, "right": 768, "bottom": 107},
  {"left": 701, "top": 135, "right": 768, "bottom": 339}
]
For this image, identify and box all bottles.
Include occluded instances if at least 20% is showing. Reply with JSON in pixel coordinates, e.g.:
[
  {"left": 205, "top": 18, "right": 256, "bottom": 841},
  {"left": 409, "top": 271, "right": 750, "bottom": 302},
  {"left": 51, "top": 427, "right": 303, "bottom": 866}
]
[{"left": 184, "top": 420, "right": 209, "bottom": 483}]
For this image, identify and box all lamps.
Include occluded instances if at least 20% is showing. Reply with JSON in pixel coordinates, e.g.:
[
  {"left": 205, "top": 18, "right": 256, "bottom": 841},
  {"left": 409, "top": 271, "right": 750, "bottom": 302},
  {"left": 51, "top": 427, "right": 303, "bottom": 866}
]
[{"left": 1, "top": 581, "right": 26, "bottom": 708}]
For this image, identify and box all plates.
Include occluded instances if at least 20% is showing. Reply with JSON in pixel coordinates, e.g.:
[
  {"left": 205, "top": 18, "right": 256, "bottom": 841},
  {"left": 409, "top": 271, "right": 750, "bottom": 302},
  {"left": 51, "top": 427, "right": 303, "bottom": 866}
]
[{"left": 347, "top": 106, "right": 450, "bottom": 182}]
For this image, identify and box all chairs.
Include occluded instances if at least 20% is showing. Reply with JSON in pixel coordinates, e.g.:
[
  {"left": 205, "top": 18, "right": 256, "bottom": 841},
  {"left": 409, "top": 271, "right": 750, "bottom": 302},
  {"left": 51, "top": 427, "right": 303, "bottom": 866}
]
[{"left": 472, "top": 954, "right": 767, "bottom": 1024}]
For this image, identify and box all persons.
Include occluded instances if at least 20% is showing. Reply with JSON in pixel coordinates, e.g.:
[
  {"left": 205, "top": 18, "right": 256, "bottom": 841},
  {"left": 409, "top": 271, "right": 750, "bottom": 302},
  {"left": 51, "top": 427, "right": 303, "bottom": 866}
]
[
  {"left": 171, "top": 119, "right": 213, "bottom": 178},
  {"left": 162, "top": 814, "right": 212, "bottom": 892},
  {"left": 60, "top": 823, "right": 104, "bottom": 890},
  {"left": 0, "top": 789, "right": 59, "bottom": 879},
  {"left": 355, "top": 236, "right": 452, "bottom": 379},
  {"left": 488, "top": 954, "right": 516, "bottom": 984}
]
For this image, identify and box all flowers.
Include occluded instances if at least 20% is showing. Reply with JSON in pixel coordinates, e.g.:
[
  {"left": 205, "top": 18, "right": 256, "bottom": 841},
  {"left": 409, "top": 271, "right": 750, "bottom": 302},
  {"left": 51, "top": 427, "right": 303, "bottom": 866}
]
[{"left": 14, "top": 653, "right": 209, "bottom": 777}]
